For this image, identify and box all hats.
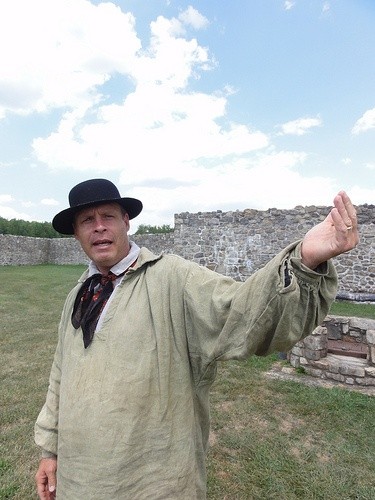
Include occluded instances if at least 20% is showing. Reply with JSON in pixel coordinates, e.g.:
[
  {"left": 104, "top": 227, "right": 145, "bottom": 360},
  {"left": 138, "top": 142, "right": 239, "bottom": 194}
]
[{"left": 52, "top": 179, "right": 143, "bottom": 235}]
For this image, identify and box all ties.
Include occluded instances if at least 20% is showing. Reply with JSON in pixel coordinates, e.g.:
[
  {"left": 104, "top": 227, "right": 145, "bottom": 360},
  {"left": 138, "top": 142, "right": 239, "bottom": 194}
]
[{"left": 71, "top": 259, "right": 137, "bottom": 348}]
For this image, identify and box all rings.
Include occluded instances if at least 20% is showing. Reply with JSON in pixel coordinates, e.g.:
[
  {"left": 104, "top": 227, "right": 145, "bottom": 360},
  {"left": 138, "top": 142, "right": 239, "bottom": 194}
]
[{"left": 348, "top": 225, "right": 353, "bottom": 230}]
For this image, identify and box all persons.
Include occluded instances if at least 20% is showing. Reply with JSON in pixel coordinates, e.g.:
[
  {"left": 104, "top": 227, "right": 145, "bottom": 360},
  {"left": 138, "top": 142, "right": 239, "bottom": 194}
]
[{"left": 34, "top": 178, "right": 359, "bottom": 500}]
